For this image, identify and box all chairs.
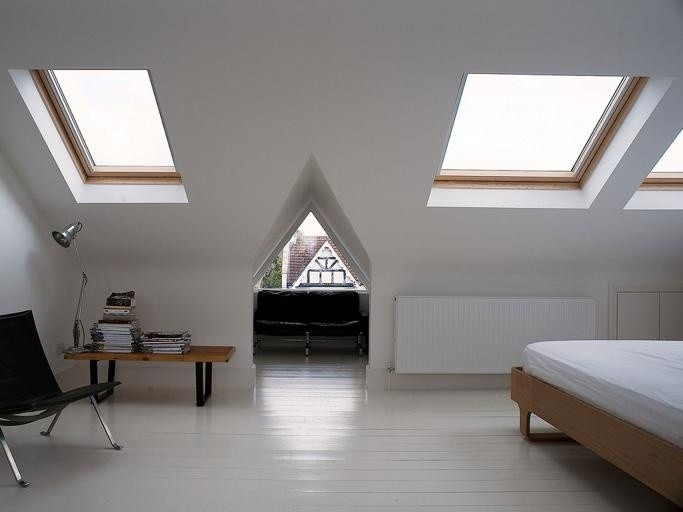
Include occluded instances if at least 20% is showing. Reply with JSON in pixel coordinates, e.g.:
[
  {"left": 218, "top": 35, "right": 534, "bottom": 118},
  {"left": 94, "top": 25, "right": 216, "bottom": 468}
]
[{"left": 0, "top": 309, "right": 124, "bottom": 488}]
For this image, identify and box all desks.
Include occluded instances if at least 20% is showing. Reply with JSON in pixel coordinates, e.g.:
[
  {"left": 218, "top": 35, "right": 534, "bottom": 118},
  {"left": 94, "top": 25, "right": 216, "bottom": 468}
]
[{"left": 63, "top": 344, "right": 237, "bottom": 407}]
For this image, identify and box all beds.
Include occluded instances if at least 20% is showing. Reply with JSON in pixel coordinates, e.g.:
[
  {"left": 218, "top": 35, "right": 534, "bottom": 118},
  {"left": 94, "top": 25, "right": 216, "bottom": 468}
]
[{"left": 507, "top": 339, "right": 683, "bottom": 510}]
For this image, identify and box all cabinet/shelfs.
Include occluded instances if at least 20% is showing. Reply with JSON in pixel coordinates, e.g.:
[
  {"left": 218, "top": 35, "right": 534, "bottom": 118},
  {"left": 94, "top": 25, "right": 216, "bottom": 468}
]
[{"left": 608, "top": 283, "right": 682, "bottom": 341}]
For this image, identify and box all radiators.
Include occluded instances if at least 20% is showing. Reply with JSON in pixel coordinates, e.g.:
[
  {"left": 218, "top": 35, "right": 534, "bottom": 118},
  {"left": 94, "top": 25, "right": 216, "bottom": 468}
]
[{"left": 388, "top": 293, "right": 599, "bottom": 376}]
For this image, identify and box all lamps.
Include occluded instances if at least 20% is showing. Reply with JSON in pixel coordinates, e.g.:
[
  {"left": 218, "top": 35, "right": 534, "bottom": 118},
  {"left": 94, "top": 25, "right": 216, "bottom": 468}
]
[{"left": 48, "top": 221, "right": 95, "bottom": 354}]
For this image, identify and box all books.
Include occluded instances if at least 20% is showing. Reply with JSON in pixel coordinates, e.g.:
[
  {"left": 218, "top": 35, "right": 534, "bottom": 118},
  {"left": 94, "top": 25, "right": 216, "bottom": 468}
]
[
  {"left": 87, "top": 322, "right": 193, "bottom": 355},
  {"left": 98, "top": 290, "right": 140, "bottom": 325}
]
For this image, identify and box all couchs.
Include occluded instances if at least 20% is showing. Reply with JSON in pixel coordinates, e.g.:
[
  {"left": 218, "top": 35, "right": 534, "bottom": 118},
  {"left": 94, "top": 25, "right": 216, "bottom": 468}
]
[{"left": 252, "top": 289, "right": 368, "bottom": 358}]
